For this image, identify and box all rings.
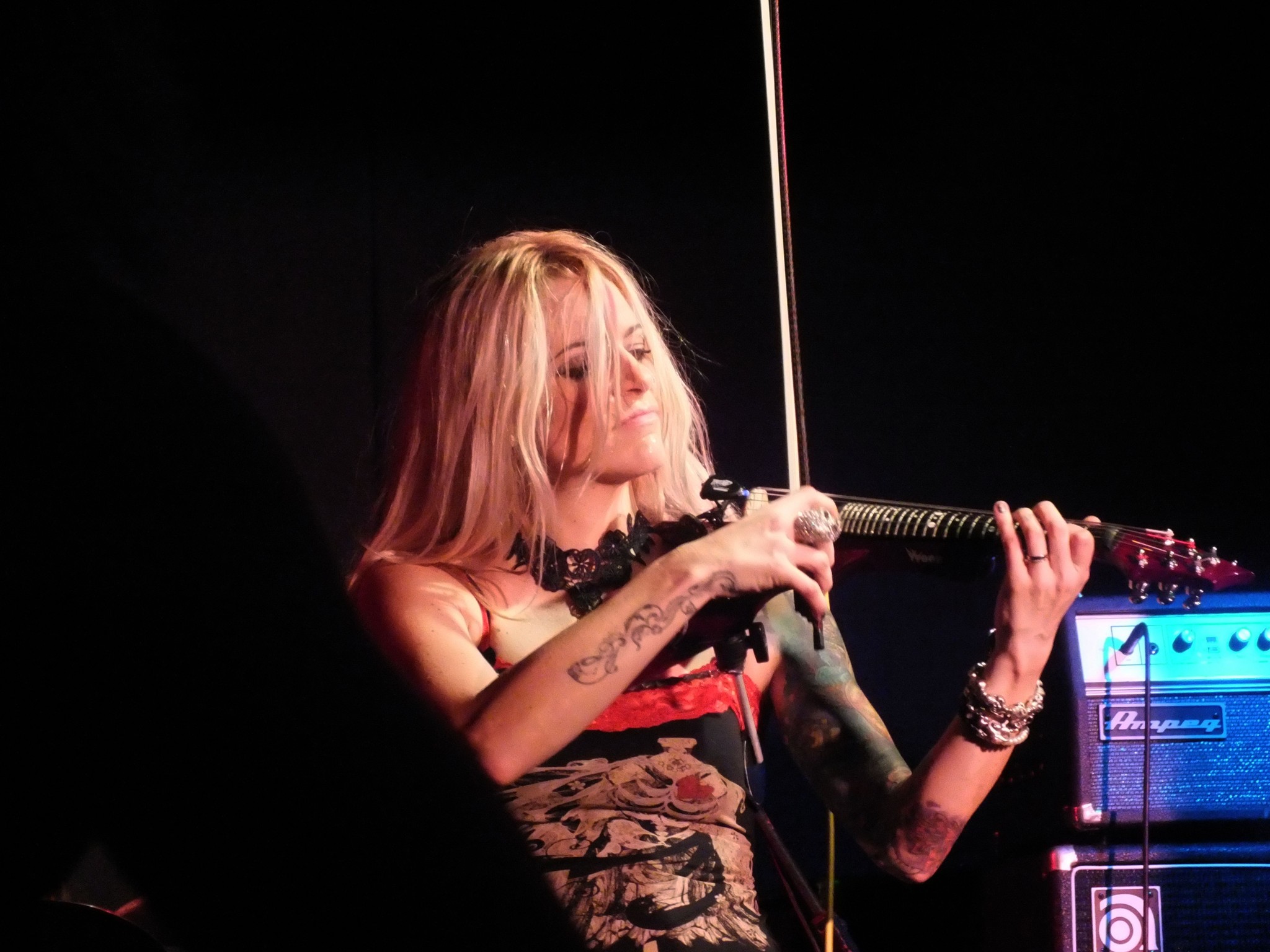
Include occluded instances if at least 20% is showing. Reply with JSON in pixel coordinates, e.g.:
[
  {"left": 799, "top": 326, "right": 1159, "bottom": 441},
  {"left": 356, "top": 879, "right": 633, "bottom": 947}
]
[
  {"left": 795, "top": 508, "right": 839, "bottom": 544},
  {"left": 1026, "top": 554, "right": 1049, "bottom": 561}
]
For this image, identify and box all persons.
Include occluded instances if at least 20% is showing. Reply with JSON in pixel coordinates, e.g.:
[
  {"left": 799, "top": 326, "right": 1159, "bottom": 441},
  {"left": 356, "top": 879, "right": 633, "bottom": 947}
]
[{"left": 350, "top": 231, "right": 1100, "bottom": 952}]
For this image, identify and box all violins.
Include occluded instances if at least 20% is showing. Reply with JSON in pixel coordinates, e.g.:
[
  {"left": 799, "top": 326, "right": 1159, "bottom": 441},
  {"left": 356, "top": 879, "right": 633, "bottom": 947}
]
[{"left": 575, "top": 472, "right": 1262, "bottom": 661}]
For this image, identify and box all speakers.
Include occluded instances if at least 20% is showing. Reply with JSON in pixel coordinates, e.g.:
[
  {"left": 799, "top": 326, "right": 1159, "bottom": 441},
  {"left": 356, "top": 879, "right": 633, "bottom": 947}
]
[
  {"left": 1050, "top": 838, "right": 1270, "bottom": 952},
  {"left": 1077, "top": 693, "right": 1270, "bottom": 825}
]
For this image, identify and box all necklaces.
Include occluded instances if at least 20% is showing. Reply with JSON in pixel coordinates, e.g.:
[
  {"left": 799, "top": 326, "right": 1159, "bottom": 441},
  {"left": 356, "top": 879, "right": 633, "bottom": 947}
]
[{"left": 506, "top": 510, "right": 654, "bottom": 618}]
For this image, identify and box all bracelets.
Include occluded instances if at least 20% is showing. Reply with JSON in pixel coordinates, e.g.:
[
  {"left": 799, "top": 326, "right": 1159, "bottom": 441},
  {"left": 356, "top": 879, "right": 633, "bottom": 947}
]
[{"left": 959, "top": 659, "right": 1045, "bottom": 746}]
